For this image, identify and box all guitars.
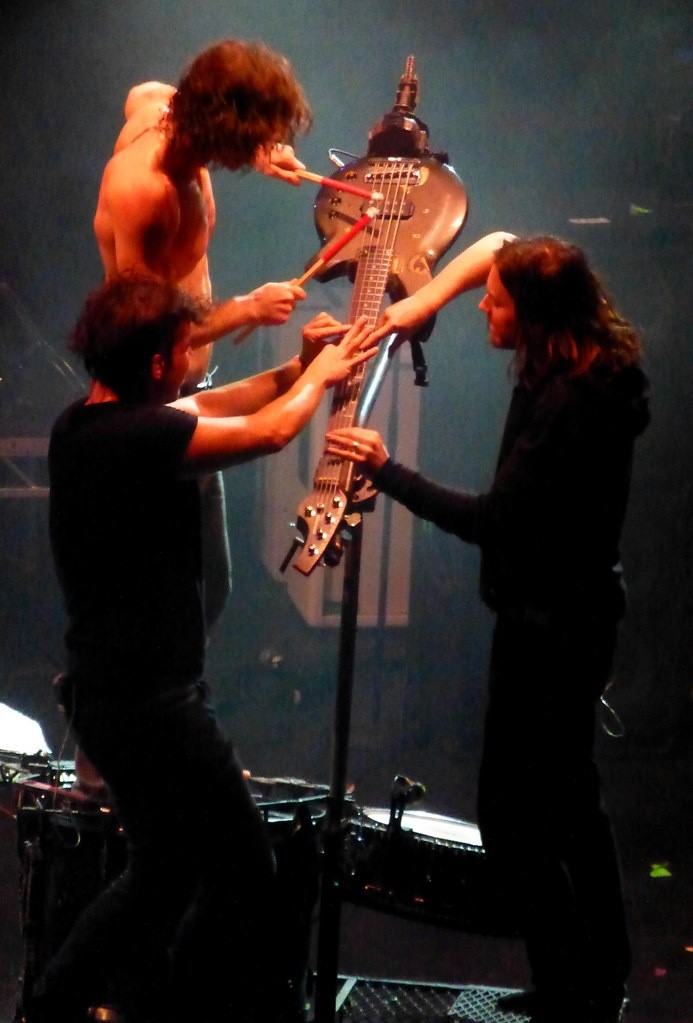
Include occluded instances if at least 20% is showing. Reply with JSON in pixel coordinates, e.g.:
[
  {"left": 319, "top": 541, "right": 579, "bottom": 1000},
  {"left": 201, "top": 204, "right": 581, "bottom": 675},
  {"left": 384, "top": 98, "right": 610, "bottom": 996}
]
[{"left": 276, "top": 55, "right": 469, "bottom": 576}]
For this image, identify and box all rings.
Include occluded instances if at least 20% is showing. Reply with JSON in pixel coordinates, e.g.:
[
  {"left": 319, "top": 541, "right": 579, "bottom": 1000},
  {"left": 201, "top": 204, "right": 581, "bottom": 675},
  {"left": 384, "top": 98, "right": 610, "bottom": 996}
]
[{"left": 353, "top": 440, "right": 359, "bottom": 450}]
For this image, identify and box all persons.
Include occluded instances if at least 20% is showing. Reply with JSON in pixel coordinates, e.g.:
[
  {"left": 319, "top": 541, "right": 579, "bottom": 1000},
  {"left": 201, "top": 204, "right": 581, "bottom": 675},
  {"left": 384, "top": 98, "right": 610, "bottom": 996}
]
[
  {"left": 324, "top": 230, "right": 657, "bottom": 1022},
  {"left": 49, "top": 268, "right": 381, "bottom": 1023},
  {"left": 53, "top": 39, "right": 308, "bottom": 793}
]
